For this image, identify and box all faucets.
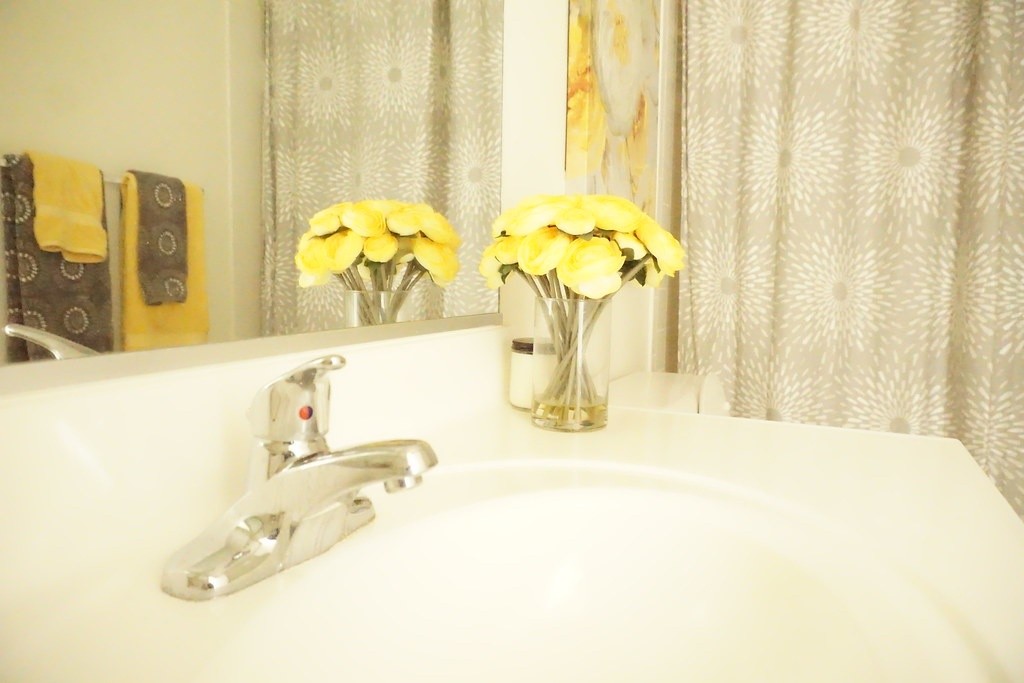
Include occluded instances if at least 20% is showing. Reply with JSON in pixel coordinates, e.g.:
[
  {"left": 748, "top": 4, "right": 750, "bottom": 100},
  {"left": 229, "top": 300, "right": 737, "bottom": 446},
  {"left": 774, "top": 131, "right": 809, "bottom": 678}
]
[{"left": 159, "top": 435, "right": 439, "bottom": 605}]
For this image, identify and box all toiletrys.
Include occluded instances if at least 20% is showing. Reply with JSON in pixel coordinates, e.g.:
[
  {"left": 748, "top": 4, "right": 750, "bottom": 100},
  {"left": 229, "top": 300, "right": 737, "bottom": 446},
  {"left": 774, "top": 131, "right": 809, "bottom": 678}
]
[{"left": 507, "top": 338, "right": 561, "bottom": 413}]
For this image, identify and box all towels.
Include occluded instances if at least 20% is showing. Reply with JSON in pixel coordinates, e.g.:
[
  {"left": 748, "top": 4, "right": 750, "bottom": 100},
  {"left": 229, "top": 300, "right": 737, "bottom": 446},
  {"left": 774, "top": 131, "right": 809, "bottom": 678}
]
[
  {"left": 27, "top": 148, "right": 110, "bottom": 263},
  {"left": 129, "top": 169, "right": 195, "bottom": 306},
  {"left": 118, "top": 168, "right": 213, "bottom": 354},
  {"left": 0, "top": 150, "right": 117, "bottom": 363}
]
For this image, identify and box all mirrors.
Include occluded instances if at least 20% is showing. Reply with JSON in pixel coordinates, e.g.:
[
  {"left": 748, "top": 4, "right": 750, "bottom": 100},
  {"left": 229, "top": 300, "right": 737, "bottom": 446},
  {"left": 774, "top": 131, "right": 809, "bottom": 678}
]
[{"left": 0, "top": 0, "right": 502, "bottom": 386}]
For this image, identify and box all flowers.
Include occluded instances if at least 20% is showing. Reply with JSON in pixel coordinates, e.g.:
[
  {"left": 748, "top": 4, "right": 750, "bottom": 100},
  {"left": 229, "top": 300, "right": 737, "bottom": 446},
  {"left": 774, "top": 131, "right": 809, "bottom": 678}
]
[
  {"left": 291, "top": 197, "right": 460, "bottom": 289},
  {"left": 477, "top": 189, "right": 689, "bottom": 301}
]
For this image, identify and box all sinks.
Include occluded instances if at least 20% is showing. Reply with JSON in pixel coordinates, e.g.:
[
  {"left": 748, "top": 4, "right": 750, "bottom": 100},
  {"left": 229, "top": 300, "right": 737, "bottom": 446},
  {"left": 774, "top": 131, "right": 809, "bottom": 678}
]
[{"left": 153, "top": 474, "right": 1021, "bottom": 683}]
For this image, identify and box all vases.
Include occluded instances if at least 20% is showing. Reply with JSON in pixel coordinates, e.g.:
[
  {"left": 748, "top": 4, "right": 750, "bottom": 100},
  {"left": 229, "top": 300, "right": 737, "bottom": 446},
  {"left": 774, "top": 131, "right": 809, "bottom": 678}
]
[
  {"left": 342, "top": 290, "right": 411, "bottom": 328},
  {"left": 529, "top": 296, "right": 612, "bottom": 433}
]
